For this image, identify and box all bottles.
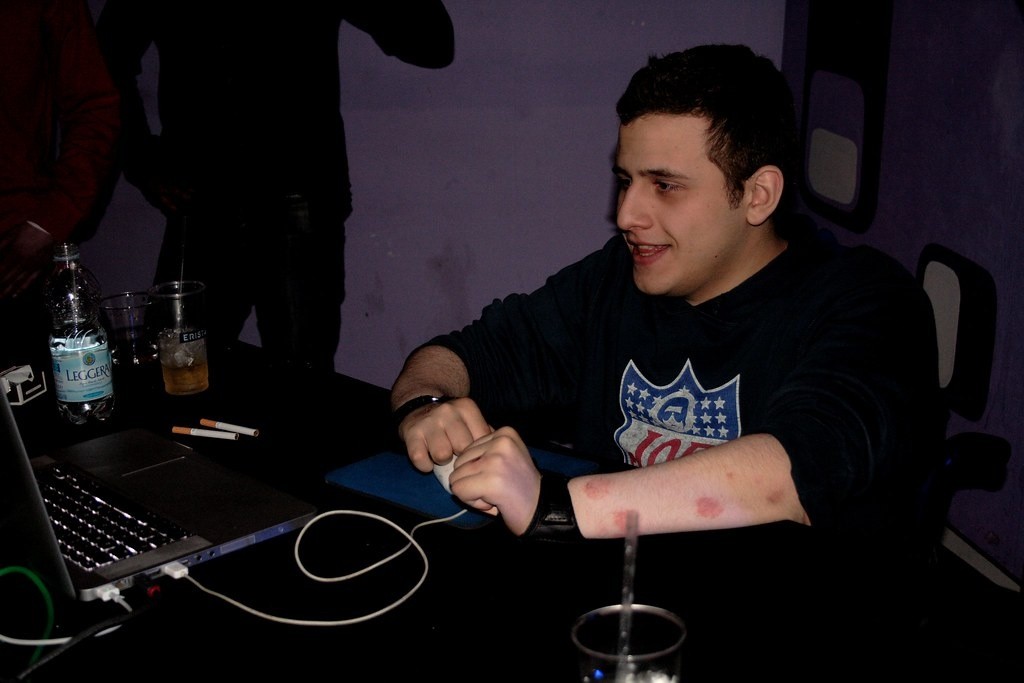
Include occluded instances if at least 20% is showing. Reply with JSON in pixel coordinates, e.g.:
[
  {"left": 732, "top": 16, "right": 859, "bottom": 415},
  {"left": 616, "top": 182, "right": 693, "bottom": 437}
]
[{"left": 46, "top": 243, "right": 116, "bottom": 424}]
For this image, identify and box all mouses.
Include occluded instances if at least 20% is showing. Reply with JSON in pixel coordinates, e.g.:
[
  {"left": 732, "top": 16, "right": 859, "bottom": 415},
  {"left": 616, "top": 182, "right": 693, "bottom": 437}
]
[{"left": 431, "top": 453, "right": 459, "bottom": 495}]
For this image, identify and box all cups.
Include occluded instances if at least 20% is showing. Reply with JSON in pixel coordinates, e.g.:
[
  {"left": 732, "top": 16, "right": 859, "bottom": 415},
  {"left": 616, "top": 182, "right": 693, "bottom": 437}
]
[
  {"left": 573, "top": 603, "right": 688, "bottom": 683},
  {"left": 149, "top": 280, "right": 209, "bottom": 395},
  {"left": 99, "top": 292, "right": 155, "bottom": 365}
]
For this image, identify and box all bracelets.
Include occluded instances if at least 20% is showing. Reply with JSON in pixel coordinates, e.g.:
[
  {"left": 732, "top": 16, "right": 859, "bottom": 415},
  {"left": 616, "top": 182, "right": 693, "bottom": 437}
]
[
  {"left": 518, "top": 468, "right": 588, "bottom": 544},
  {"left": 394, "top": 396, "right": 446, "bottom": 428}
]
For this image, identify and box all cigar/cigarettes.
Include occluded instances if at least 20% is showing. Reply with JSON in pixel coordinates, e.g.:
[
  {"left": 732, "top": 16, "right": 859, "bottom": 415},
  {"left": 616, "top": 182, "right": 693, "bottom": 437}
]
[
  {"left": 200, "top": 418, "right": 259, "bottom": 436},
  {"left": 173, "top": 426, "right": 238, "bottom": 441}
]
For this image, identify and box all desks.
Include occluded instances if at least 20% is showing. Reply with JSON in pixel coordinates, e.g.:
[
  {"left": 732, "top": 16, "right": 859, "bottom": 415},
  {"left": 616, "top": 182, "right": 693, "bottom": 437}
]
[{"left": 0, "top": 339, "right": 1023, "bottom": 683}]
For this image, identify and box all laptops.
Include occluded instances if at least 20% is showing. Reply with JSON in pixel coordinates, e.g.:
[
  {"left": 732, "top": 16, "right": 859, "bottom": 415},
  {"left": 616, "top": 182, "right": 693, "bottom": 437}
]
[{"left": 0, "top": 382, "right": 315, "bottom": 601}]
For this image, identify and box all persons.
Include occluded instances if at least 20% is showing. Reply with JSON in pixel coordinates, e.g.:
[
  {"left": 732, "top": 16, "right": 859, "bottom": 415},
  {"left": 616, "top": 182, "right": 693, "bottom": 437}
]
[
  {"left": 96, "top": 0, "right": 453, "bottom": 371},
  {"left": 391, "top": 42, "right": 938, "bottom": 565},
  {"left": 0, "top": 1, "right": 122, "bottom": 302}
]
